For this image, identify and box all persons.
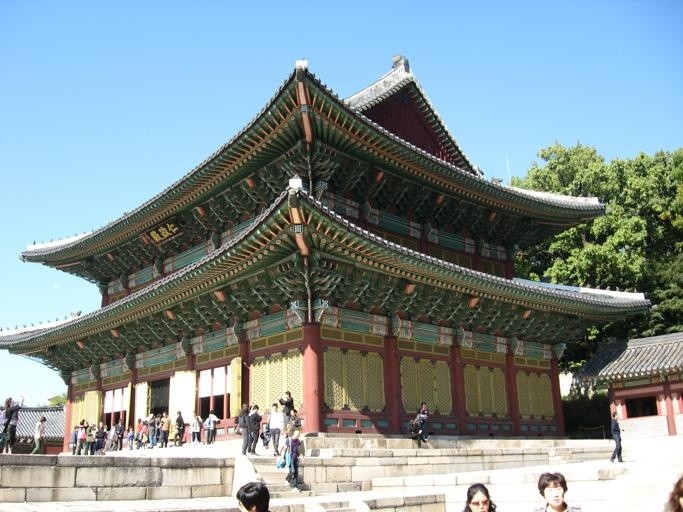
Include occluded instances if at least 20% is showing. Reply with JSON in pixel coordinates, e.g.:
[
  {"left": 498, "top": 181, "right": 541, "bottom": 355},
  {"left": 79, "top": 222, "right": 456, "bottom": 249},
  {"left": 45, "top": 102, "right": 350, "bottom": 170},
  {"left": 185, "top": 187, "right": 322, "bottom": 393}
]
[
  {"left": 407, "top": 416, "right": 426, "bottom": 444},
  {"left": 663, "top": 474, "right": 683, "bottom": 512},
  {"left": 31, "top": 418, "right": 46, "bottom": 454},
  {"left": 72, "top": 409, "right": 221, "bottom": 455},
  {"left": 1, "top": 395, "right": 24, "bottom": 454},
  {"left": 418, "top": 403, "right": 430, "bottom": 440},
  {"left": 610, "top": 411, "right": 624, "bottom": 463},
  {"left": 533, "top": 472, "right": 581, "bottom": 512},
  {"left": 234, "top": 391, "right": 302, "bottom": 492},
  {"left": 237, "top": 482, "right": 273, "bottom": 512},
  {"left": 463, "top": 483, "right": 496, "bottom": 512}
]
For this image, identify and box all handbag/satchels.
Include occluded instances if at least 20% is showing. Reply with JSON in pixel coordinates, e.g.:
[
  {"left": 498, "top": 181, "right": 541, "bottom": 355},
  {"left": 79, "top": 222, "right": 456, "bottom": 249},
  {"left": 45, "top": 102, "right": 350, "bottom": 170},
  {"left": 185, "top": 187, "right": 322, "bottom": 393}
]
[
  {"left": 0, "top": 409, "right": 8, "bottom": 425},
  {"left": 285, "top": 451, "right": 291, "bottom": 466}
]
